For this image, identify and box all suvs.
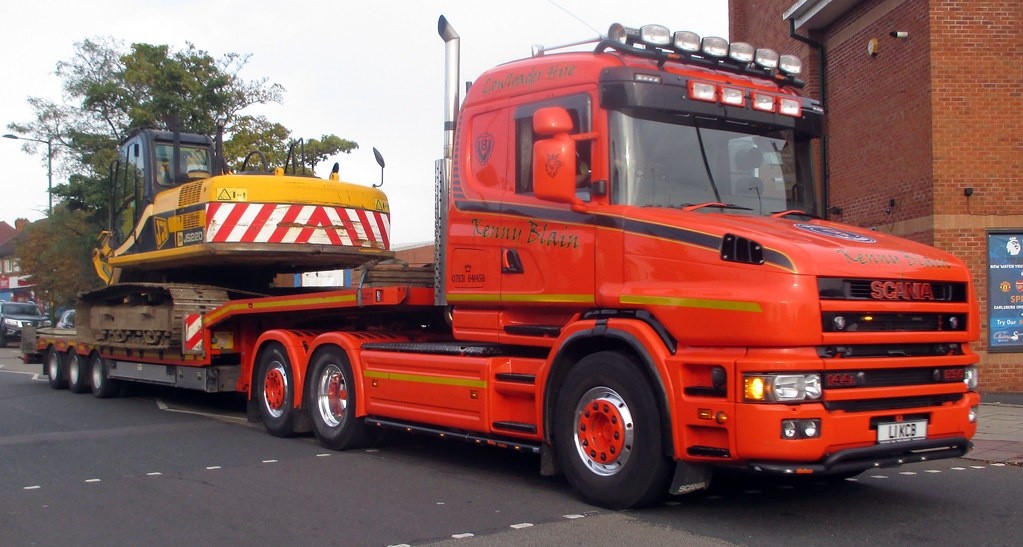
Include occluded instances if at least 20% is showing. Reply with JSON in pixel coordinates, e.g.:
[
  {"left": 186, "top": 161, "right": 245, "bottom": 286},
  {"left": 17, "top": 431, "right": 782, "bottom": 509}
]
[{"left": 0, "top": 300, "right": 52, "bottom": 350}]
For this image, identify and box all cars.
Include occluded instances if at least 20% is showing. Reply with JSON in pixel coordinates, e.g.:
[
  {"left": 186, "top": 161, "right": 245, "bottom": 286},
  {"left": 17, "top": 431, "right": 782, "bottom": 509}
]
[{"left": 56, "top": 309, "right": 76, "bottom": 330}]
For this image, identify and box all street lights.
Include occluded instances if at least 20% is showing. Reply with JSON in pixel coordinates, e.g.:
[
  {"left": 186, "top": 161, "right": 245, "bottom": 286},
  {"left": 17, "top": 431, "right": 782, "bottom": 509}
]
[{"left": 2, "top": 132, "right": 53, "bottom": 216}]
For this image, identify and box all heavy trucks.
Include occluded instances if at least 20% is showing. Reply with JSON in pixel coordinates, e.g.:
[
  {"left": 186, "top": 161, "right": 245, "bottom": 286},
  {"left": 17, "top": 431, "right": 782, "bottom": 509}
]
[{"left": 23, "top": 22, "right": 982, "bottom": 511}]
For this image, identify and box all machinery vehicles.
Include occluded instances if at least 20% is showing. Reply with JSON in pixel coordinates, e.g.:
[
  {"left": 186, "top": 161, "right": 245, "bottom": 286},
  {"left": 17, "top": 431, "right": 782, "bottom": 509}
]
[{"left": 71, "top": 110, "right": 398, "bottom": 356}]
